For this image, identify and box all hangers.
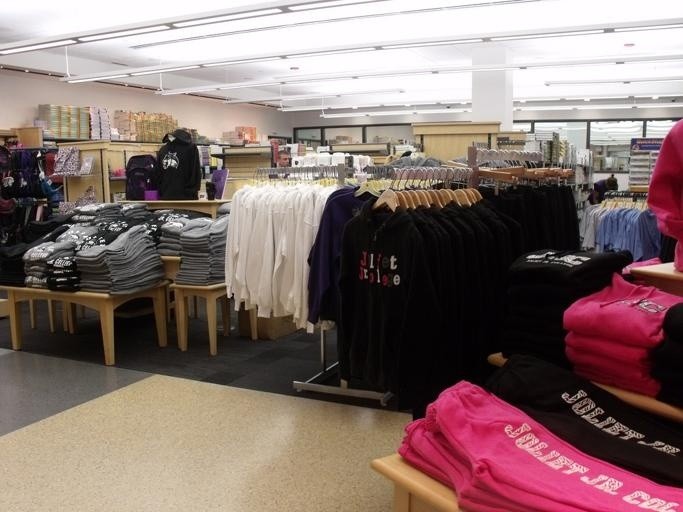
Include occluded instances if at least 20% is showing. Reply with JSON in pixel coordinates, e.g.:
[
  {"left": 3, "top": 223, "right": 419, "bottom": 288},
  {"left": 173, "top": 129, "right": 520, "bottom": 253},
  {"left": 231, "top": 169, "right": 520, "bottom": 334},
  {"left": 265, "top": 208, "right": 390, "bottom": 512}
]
[
  {"left": 372, "top": 166, "right": 482, "bottom": 212},
  {"left": 248, "top": 165, "right": 338, "bottom": 187},
  {"left": 476, "top": 149, "right": 573, "bottom": 196},
  {"left": 601, "top": 191, "right": 649, "bottom": 212}
]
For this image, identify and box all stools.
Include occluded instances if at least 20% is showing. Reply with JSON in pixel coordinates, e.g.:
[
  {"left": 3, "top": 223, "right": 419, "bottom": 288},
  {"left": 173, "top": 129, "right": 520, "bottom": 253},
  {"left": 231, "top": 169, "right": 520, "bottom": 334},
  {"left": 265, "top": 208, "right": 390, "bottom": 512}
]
[{"left": 171, "top": 283, "right": 258, "bottom": 357}]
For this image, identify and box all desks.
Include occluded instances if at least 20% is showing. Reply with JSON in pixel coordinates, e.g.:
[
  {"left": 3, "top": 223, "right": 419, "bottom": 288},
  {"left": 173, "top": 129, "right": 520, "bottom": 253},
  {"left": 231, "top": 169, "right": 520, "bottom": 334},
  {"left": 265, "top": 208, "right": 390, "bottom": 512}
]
[
  {"left": 593, "top": 381, "right": 682, "bottom": 420},
  {"left": 631, "top": 261, "right": 683, "bottom": 291},
  {"left": 369, "top": 451, "right": 462, "bottom": 512},
  {"left": 117, "top": 197, "right": 232, "bottom": 219}
]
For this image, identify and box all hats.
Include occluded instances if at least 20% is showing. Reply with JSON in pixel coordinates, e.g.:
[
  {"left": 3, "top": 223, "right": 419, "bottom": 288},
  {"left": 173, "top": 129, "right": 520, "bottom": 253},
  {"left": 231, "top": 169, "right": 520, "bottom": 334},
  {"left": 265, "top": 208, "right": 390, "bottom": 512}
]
[{"left": 162, "top": 128, "right": 193, "bottom": 144}]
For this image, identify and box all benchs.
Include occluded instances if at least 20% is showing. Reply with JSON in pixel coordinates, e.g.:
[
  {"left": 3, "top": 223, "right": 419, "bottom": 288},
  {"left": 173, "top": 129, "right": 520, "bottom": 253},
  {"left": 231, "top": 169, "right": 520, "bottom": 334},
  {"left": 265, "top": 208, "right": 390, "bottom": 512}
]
[{"left": 0, "top": 279, "right": 168, "bottom": 365}]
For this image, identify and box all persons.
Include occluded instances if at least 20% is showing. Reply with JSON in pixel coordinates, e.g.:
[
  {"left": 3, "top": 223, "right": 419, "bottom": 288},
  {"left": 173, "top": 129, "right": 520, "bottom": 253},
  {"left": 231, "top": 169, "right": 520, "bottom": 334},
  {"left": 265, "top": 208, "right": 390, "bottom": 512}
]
[
  {"left": 270, "top": 150, "right": 291, "bottom": 178},
  {"left": 588, "top": 174, "right": 619, "bottom": 205}
]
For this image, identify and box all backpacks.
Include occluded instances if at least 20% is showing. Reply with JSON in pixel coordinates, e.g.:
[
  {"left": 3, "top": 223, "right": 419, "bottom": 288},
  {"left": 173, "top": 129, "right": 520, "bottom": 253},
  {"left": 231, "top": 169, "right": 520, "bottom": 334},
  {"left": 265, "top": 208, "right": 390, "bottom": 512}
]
[{"left": 125, "top": 155, "right": 157, "bottom": 201}]
